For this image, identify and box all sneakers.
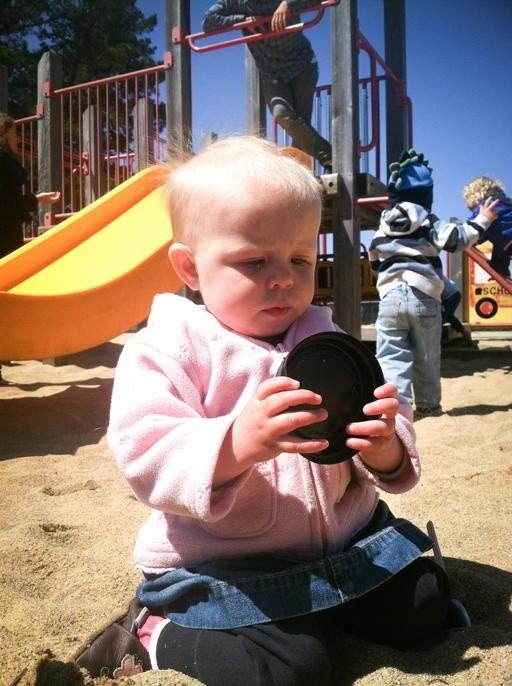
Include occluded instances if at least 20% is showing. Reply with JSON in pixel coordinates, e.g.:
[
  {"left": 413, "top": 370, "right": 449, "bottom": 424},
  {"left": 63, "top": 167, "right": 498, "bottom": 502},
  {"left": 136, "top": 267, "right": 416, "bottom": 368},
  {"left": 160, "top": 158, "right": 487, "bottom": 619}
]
[
  {"left": 416, "top": 406, "right": 441, "bottom": 417},
  {"left": 67, "top": 598, "right": 152, "bottom": 677}
]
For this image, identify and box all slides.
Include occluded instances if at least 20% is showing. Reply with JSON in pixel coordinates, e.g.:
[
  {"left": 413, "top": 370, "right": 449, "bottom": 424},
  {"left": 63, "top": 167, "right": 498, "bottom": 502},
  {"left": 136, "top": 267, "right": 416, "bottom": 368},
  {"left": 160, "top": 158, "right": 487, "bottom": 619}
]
[{"left": 0, "top": 147, "right": 315, "bottom": 358}]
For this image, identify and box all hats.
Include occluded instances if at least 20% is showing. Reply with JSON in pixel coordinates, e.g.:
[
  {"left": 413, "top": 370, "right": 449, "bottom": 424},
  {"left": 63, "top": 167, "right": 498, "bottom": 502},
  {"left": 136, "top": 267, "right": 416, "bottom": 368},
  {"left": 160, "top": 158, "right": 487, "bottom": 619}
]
[{"left": 387, "top": 149, "right": 433, "bottom": 208}]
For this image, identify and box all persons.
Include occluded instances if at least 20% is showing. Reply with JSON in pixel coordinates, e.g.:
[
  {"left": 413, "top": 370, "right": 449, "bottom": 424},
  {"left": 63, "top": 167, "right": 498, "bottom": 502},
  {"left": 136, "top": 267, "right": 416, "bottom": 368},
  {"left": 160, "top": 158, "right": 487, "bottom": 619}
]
[
  {"left": 202, "top": 0, "right": 333, "bottom": 176},
  {"left": 439, "top": 275, "right": 471, "bottom": 344},
  {"left": 0, "top": 112, "right": 61, "bottom": 259},
  {"left": 463, "top": 174, "right": 511, "bottom": 282},
  {"left": 368, "top": 151, "right": 500, "bottom": 423},
  {"left": 67, "top": 138, "right": 451, "bottom": 686}
]
